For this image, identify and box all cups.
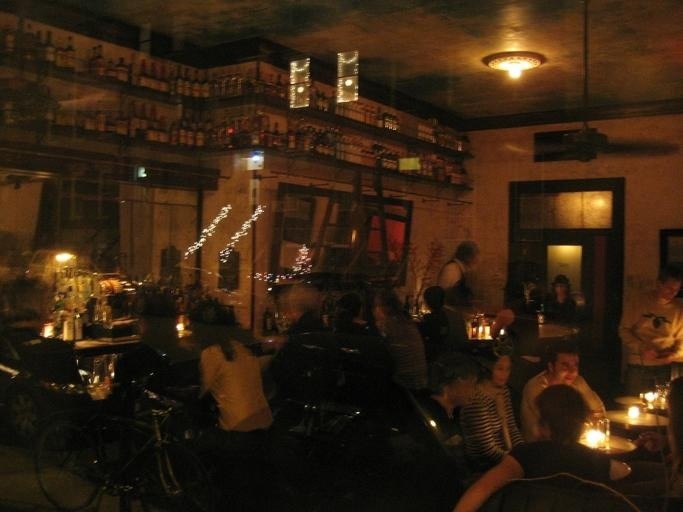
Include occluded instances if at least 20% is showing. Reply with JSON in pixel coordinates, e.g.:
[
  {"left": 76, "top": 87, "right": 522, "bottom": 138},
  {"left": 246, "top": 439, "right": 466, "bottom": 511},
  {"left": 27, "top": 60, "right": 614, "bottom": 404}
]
[{"left": 536, "top": 310, "right": 545, "bottom": 325}]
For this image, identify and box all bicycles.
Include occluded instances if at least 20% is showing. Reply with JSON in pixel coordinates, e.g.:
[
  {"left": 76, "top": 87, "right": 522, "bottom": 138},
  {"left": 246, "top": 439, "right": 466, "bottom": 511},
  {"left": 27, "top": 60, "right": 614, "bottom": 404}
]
[{"left": 34, "top": 384, "right": 211, "bottom": 510}]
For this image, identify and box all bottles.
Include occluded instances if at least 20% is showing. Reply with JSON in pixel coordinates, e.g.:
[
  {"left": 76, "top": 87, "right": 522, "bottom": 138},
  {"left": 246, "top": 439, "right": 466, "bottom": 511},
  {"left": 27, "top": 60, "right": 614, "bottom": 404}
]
[
  {"left": 470, "top": 317, "right": 479, "bottom": 337},
  {"left": 101, "top": 301, "right": 112, "bottom": 330},
  {"left": 583, "top": 410, "right": 612, "bottom": 450},
  {"left": 0, "top": 17, "right": 259, "bottom": 152},
  {"left": 260, "top": 65, "right": 472, "bottom": 186},
  {"left": 91, "top": 299, "right": 101, "bottom": 325},
  {"left": 262, "top": 305, "right": 273, "bottom": 337},
  {"left": 38, "top": 305, "right": 82, "bottom": 341}
]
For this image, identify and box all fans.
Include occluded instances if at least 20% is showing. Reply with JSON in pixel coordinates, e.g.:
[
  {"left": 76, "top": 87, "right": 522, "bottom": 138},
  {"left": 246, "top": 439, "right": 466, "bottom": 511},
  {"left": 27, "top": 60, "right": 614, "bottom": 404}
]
[{"left": 500, "top": 0, "right": 680, "bottom": 163}]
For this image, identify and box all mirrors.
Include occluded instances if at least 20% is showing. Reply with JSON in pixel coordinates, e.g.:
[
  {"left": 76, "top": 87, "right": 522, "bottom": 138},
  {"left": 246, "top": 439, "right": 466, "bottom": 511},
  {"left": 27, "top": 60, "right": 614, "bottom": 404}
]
[{"left": 268, "top": 182, "right": 414, "bottom": 291}]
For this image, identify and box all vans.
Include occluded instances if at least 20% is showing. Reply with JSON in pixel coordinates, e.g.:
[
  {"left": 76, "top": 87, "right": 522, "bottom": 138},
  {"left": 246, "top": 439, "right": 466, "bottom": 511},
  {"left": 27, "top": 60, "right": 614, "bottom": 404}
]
[{"left": 21, "top": 247, "right": 107, "bottom": 290}]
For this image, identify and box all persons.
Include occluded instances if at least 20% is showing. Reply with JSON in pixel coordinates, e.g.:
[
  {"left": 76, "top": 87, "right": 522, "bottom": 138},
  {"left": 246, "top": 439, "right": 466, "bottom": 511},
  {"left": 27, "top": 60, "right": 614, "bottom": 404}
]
[
  {"left": 617, "top": 265, "right": 683, "bottom": 395},
  {"left": 490, "top": 283, "right": 544, "bottom": 365},
  {"left": 542, "top": 275, "right": 576, "bottom": 324},
  {"left": 402, "top": 341, "right": 631, "bottom": 512},
  {"left": 0, "top": 275, "right": 94, "bottom": 428},
  {"left": 436, "top": 240, "right": 479, "bottom": 295},
  {"left": 110, "top": 289, "right": 470, "bottom": 455},
  {"left": 639, "top": 375, "right": 683, "bottom": 512}
]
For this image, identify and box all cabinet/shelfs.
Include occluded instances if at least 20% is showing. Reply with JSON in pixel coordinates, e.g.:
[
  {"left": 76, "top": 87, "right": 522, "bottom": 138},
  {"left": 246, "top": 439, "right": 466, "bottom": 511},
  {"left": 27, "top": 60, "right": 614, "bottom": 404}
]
[{"left": 0, "top": 53, "right": 474, "bottom": 200}]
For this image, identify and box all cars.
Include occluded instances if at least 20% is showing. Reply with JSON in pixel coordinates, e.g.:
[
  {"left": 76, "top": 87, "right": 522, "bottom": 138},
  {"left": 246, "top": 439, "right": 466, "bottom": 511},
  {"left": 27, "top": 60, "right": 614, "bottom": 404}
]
[{"left": 0, "top": 305, "right": 95, "bottom": 440}]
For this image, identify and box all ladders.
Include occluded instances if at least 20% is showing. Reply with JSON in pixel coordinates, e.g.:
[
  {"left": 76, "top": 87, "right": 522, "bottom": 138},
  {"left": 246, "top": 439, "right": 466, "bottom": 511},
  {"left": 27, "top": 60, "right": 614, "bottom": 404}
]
[
  {"left": 312, "top": 195, "right": 357, "bottom": 269},
  {"left": 354, "top": 160, "right": 389, "bottom": 320}
]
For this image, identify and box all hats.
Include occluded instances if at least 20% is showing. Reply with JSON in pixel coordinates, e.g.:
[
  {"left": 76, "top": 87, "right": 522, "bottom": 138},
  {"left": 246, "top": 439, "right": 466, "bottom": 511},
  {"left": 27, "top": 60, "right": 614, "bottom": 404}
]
[{"left": 552, "top": 275, "right": 568, "bottom": 286}]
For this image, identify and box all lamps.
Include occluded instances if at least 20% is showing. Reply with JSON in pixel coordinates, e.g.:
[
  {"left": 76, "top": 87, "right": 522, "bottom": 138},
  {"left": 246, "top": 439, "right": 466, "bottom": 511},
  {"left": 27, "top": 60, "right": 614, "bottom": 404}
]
[{"left": 481, "top": 34, "right": 547, "bottom": 81}]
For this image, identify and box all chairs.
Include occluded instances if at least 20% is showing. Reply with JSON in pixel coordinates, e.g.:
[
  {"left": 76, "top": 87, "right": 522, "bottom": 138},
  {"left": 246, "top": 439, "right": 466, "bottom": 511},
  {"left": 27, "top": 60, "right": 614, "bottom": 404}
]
[
  {"left": 572, "top": 291, "right": 588, "bottom": 323},
  {"left": 497, "top": 471, "right": 640, "bottom": 512}
]
